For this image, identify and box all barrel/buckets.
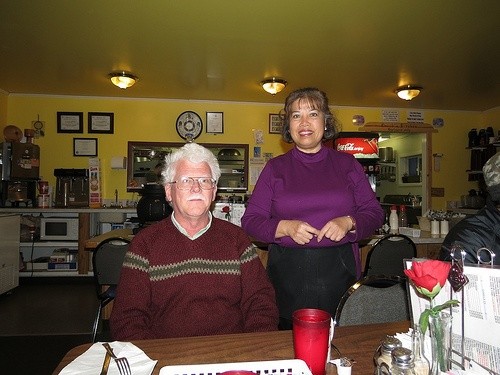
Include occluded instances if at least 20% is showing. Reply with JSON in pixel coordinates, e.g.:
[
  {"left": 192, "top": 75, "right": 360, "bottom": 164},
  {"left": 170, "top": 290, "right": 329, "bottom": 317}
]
[{"left": 379, "top": 147, "right": 393, "bottom": 163}]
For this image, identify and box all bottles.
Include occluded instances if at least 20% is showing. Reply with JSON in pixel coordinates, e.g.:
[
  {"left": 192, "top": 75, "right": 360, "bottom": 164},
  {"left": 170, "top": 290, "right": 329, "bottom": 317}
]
[
  {"left": 468, "top": 127, "right": 495, "bottom": 147},
  {"left": 377, "top": 338, "right": 402, "bottom": 374},
  {"left": 37, "top": 194, "right": 50, "bottom": 209},
  {"left": 391, "top": 348, "right": 414, "bottom": 375},
  {"left": 399, "top": 205, "right": 407, "bottom": 228},
  {"left": 28, "top": 224, "right": 36, "bottom": 242},
  {"left": 409, "top": 330, "right": 430, "bottom": 375},
  {"left": 469, "top": 188, "right": 488, "bottom": 208},
  {"left": 389, "top": 205, "right": 399, "bottom": 230},
  {"left": 429, "top": 218, "right": 449, "bottom": 234}
]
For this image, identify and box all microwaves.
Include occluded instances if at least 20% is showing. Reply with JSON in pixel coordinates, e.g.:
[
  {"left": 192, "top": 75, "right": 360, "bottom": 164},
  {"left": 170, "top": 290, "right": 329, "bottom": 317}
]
[{"left": 40, "top": 217, "right": 79, "bottom": 242}]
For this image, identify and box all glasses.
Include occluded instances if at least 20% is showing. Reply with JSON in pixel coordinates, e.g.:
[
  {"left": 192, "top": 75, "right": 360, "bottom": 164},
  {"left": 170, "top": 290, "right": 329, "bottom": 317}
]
[{"left": 169, "top": 176, "right": 216, "bottom": 190}]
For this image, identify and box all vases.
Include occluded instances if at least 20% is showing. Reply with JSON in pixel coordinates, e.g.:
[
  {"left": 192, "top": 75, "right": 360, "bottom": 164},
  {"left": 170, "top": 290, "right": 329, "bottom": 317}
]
[
  {"left": 429, "top": 312, "right": 453, "bottom": 375},
  {"left": 441, "top": 221, "right": 449, "bottom": 234},
  {"left": 431, "top": 221, "right": 439, "bottom": 234}
]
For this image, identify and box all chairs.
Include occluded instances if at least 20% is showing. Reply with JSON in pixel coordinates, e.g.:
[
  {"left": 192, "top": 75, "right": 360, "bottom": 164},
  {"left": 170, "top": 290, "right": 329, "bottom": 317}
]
[
  {"left": 334, "top": 235, "right": 418, "bottom": 327},
  {"left": 91, "top": 237, "right": 132, "bottom": 345}
]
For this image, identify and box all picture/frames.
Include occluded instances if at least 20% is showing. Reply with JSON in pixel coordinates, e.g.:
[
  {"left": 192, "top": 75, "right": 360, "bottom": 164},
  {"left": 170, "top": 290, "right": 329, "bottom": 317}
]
[
  {"left": 73, "top": 138, "right": 98, "bottom": 157},
  {"left": 88, "top": 111, "right": 114, "bottom": 134},
  {"left": 268, "top": 113, "right": 284, "bottom": 134},
  {"left": 206, "top": 112, "right": 223, "bottom": 133},
  {"left": 56, "top": 112, "right": 84, "bottom": 134}
]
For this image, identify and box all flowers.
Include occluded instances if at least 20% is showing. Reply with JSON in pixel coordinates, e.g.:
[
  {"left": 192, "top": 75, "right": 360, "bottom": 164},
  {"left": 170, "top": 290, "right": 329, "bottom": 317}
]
[
  {"left": 439, "top": 211, "right": 452, "bottom": 221},
  {"left": 426, "top": 210, "right": 438, "bottom": 221},
  {"left": 222, "top": 206, "right": 234, "bottom": 221},
  {"left": 402, "top": 260, "right": 460, "bottom": 334}
]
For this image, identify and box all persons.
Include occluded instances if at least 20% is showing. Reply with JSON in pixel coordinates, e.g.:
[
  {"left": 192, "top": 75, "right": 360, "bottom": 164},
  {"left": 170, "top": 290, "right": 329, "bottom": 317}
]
[
  {"left": 109, "top": 144, "right": 278, "bottom": 341},
  {"left": 438, "top": 152, "right": 500, "bottom": 265},
  {"left": 241, "top": 87, "right": 387, "bottom": 331}
]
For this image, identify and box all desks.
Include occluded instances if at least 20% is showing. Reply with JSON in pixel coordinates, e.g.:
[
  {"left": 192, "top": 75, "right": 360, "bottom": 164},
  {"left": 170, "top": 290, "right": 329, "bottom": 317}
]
[
  {"left": 353, "top": 230, "right": 445, "bottom": 273},
  {"left": 53, "top": 325, "right": 415, "bottom": 375},
  {"left": 84, "top": 227, "right": 137, "bottom": 250}
]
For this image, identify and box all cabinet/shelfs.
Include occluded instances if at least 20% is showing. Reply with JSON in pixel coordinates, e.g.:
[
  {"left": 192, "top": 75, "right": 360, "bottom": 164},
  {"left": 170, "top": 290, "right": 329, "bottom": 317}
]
[
  {"left": 126, "top": 140, "right": 249, "bottom": 193},
  {"left": 465, "top": 145, "right": 492, "bottom": 174},
  {"left": 0, "top": 208, "right": 86, "bottom": 277}
]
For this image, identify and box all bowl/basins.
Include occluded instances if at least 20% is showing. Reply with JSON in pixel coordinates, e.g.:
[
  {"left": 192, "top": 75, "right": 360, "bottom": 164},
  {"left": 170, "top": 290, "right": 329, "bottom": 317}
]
[{"left": 416, "top": 216, "right": 431, "bottom": 231}]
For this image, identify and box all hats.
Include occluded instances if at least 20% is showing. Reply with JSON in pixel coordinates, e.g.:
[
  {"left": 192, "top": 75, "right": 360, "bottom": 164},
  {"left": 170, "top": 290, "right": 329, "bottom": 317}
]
[{"left": 483, "top": 152, "right": 500, "bottom": 202}]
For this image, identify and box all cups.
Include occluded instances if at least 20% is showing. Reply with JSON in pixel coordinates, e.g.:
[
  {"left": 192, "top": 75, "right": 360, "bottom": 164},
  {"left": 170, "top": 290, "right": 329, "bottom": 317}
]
[
  {"left": 292, "top": 308, "right": 330, "bottom": 375},
  {"left": 36, "top": 180, "right": 48, "bottom": 194}
]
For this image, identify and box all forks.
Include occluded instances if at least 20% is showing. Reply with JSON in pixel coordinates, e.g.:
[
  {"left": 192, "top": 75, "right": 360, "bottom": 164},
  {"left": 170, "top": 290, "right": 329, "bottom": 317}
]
[{"left": 102, "top": 342, "right": 131, "bottom": 375}]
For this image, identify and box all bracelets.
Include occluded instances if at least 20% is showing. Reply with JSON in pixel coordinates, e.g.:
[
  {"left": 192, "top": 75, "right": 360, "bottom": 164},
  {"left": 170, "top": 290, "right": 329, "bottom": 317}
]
[{"left": 348, "top": 216, "right": 356, "bottom": 231}]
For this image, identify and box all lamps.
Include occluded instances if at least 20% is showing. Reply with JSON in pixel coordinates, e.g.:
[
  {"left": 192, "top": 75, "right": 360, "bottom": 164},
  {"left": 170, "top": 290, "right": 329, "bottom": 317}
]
[
  {"left": 258, "top": 78, "right": 288, "bottom": 96},
  {"left": 108, "top": 71, "right": 138, "bottom": 90},
  {"left": 394, "top": 84, "right": 423, "bottom": 101}
]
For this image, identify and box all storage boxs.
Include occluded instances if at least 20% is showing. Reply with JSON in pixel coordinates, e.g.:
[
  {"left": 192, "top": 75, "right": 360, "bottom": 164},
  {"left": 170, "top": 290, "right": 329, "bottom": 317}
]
[{"left": 399, "top": 227, "right": 420, "bottom": 237}]
